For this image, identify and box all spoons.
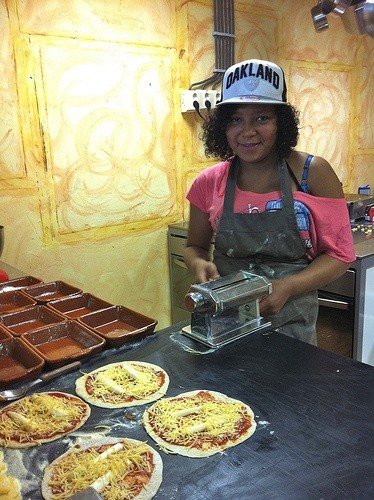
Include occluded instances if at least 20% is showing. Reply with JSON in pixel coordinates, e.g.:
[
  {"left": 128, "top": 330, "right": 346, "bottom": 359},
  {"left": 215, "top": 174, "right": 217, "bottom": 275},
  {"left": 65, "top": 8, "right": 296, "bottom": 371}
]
[{"left": 0, "top": 361, "right": 82, "bottom": 401}]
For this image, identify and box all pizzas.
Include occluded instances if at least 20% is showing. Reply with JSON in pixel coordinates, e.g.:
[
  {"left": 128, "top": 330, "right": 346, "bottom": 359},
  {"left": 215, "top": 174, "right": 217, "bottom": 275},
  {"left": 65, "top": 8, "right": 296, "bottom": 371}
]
[
  {"left": 75, "top": 360, "right": 170, "bottom": 409},
  {"left": 0, "top": 392, "right": 90, "bottom": 450},
  {"left": 142, "top": 389, "right": 257, "bottom": 459},
  {"left": 40, "top": 436, "right": 163, "bottom": 500}
]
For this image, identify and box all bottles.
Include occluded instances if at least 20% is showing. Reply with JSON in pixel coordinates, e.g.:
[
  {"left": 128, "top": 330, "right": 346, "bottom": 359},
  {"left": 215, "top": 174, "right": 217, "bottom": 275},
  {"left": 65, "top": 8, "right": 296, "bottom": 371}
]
[{"left": 364, "top": 203, "right": 374, "bottom": 230}]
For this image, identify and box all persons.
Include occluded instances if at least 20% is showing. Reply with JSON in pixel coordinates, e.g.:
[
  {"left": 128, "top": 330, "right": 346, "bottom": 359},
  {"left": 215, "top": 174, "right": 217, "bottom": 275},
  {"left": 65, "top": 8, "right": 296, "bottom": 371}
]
[{"left": 185, "top": 60, "right": 356, "bottom": 347}]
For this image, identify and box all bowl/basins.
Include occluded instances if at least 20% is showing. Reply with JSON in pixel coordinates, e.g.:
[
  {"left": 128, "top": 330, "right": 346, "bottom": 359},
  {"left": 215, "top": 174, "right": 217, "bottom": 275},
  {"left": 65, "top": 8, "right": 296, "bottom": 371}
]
[
  {"left": 20, "top": 319, "right": 106, "bottom": 370},
  {"left": 78, "top": 304, "right": 158, "bottom": 348},
  {"left": 0, "top": 275, "right": 45, "bottom": 391},
  {"left": 46, "top": 292, "right": 116, "bottom": 320},
  {"left": 21, "top": 280, "right": 83, "bottom": 305}
]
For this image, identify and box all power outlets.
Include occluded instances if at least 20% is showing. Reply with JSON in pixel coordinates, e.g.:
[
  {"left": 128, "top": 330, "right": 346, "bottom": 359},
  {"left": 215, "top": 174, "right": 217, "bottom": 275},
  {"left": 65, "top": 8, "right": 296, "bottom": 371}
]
[{"left": 181, "top": 89, "right": 220, "bottom": 113}]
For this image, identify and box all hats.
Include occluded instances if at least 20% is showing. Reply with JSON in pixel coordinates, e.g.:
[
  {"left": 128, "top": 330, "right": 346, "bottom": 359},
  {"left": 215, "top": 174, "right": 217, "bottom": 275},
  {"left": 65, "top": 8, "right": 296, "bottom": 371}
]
[{"left": 215, "top": 59, "right": 287, "bottom": 105}]
